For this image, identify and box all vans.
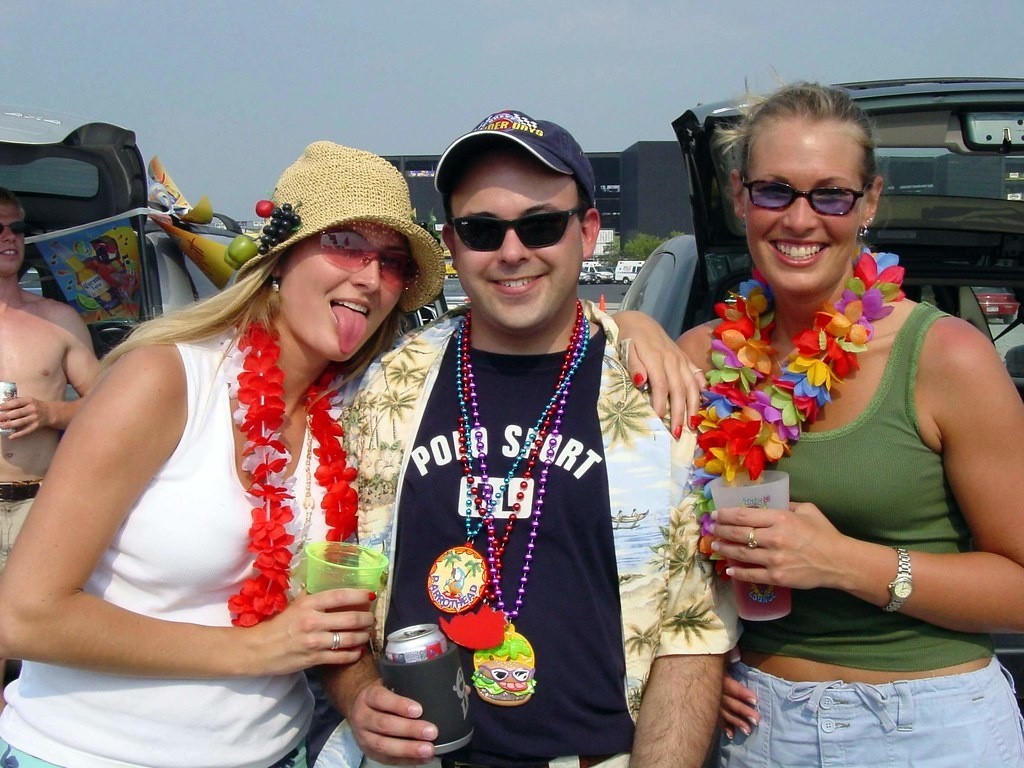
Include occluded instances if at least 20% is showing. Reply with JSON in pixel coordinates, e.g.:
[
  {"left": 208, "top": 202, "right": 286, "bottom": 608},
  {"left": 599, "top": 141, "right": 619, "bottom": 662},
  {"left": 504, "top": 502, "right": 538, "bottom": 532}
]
[{"left": 615, "top": 261, "right": 645, "bottom": 285}]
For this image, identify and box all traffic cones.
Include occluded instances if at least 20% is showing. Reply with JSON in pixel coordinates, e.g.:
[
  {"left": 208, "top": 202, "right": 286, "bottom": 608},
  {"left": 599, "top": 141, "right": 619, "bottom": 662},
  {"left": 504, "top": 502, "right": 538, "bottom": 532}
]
[{"left": 599, "top": 294, "right": 607, "bottom": 313}]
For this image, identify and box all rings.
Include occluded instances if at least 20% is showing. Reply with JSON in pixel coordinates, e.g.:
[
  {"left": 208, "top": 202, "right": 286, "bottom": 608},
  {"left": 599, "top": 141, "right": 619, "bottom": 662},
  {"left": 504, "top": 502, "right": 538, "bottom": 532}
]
[
  {"left": 693, "top": 367, "right": 704, "bottom": 375},
  {"left": 330, "top": 632, "right": 341, "bottom": 650},
  {"left": 747, "top": 527, "right": 758, "bottom": 548}
]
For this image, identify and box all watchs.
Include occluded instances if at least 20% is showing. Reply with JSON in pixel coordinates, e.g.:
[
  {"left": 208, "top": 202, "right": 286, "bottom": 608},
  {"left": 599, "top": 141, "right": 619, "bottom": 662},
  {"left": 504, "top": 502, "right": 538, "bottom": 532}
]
[{"left": 882, "top": 547, "right": 914, "bottom": 612}]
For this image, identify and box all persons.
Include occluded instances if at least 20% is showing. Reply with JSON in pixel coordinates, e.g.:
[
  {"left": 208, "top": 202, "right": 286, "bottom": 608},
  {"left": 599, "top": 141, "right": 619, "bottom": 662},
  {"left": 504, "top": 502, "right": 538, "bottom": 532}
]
[
  {"left": 677, "top": 84, "right": 1024, "bottom": 768},
  {"left": 0, "top": 187, "right": 100, "bottom": 573},
  {"left": 0, "top": 140, "right": 714, "bottom": 768},
  {"left": 322, "top": 109, "right": 745, "bottom": 768}
]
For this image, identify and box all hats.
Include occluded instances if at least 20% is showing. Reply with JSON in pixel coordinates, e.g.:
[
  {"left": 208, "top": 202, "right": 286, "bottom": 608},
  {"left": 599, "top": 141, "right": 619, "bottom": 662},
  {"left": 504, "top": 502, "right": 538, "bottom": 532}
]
[
  {"left": 435, "top": 110, "right": 597, "bottom": 211},
  {"left": 232, "top": 141, "right": 446, "bottom": 314}
]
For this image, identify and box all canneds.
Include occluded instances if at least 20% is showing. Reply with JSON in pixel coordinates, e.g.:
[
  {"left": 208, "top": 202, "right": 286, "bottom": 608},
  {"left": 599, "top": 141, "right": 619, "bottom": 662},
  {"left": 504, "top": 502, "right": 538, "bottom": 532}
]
[
  {"left": 0, "top": 380, "right": 18, "bottom": 435},
  {"left": 385, "top": 624, "right": 447, "bottom": 664}
]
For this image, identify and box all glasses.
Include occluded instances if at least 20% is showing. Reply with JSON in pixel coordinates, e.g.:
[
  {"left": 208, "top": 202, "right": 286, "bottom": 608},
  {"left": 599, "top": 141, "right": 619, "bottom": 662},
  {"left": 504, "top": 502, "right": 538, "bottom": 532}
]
[
  {"left": 316, "top": 230, "right": 421, "bottom": 294},
  {"left": 447, "top": 207, "right": 582, "bottom": 251},
  {"left": 740, "top": 177, "right": 869, "bottom": 217},
  {"left": 0, "top": 221, "right": 27, "bottom": 235}
]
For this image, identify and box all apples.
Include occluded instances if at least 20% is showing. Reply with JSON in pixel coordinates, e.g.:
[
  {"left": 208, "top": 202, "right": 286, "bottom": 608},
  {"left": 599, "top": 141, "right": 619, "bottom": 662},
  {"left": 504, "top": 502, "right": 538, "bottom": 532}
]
[
  {"left": 223, "top": 235, "right": 257, "bottom": 268},
  {"left": 255, "top": 199, "right": 275, "bottom": 218}
]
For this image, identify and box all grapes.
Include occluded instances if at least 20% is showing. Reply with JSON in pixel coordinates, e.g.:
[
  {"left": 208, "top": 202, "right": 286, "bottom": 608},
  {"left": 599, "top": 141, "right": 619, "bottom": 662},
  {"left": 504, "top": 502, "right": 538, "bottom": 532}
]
[{"left": 259, "top": 204, "right": 301, "bottom": 255}]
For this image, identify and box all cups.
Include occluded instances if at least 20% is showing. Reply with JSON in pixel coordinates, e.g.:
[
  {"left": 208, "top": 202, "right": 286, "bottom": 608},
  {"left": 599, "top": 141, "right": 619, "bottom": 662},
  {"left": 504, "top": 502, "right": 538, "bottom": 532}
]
[
  {"left": 378, "top": 643, "right": 474, "bottom": 755},
  {"left": 711, "top": 470, "right": 791, "bottom": 621},
  {"left": 305, "top": 541, "right": 389, "bottom": 650}
]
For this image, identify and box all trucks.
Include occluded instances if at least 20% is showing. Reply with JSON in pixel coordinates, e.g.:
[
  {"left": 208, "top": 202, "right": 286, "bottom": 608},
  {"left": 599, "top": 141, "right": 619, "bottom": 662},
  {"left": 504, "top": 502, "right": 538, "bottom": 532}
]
[{"left": 444, "top": 254, "right": 459, "bottom": 278}]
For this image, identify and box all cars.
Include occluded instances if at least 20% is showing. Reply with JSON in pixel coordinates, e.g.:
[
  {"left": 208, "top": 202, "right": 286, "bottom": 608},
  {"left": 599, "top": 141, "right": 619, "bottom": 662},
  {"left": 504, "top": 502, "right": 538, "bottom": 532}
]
[
  {"left": 0, "top": 106, "right": 256, "bottom": 404},
  {"left": 578, "top": 266, "right": 614, "bottom": 285},
  {"left": 970, "top": 285, "right": 1018, "bottom": 324},
  {"left": 606, "top": 267, "right": 615, "bottom": 274},
  {"left": 616, "top": 77, "right": 1024, "bottom": 718}
]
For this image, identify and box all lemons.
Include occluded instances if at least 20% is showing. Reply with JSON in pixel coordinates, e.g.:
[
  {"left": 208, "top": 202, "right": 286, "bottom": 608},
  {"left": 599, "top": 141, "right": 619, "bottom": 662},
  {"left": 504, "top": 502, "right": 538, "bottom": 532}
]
[{"left": 76, "top": 294, "right": 100, "bottom": 310}]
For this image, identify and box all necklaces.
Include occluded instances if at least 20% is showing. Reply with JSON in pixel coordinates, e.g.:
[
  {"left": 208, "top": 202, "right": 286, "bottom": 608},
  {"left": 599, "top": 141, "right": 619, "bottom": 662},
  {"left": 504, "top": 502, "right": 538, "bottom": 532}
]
[
  {"left": 426, "top": 297, "right": 592, "bottom": 707},
  {"left": 690, "top": 247, "right": 906, "bottom": 580},
  {"left": 226, "top": 320, "right": 362, "bottom": 629}
]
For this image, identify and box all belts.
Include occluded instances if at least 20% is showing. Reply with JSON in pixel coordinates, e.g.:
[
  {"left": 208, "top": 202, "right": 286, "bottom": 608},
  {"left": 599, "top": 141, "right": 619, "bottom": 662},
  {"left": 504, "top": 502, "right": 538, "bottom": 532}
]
[{"left": 0, "top": 484, "right": 43, "bottom": 502}]
